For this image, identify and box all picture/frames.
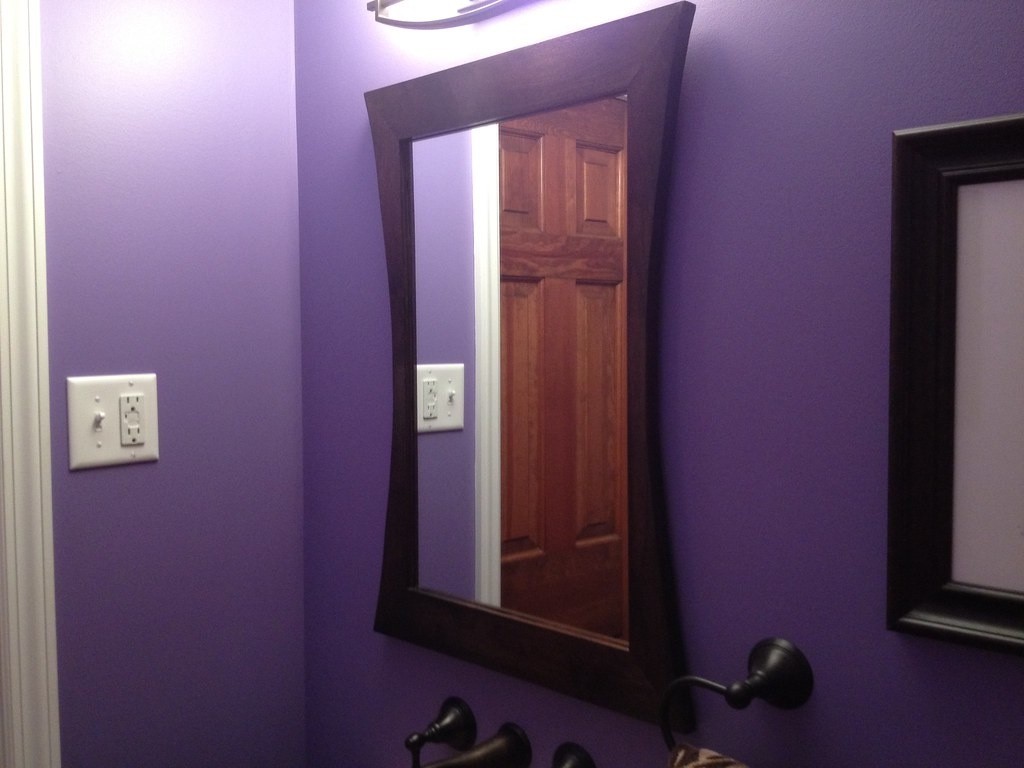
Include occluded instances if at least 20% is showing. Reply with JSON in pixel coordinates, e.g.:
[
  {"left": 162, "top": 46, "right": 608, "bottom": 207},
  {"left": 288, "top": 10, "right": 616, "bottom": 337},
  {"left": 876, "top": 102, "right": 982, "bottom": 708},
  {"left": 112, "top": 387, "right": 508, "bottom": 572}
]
[{"left": 888, "top": 115, "right": 1023, "bottom": 653}]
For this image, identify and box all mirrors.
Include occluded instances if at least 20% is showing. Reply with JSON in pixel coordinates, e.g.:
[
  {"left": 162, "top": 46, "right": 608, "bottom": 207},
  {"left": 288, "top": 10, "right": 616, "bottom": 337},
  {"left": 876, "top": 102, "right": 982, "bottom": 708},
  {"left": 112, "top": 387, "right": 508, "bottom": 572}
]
[{"left": 398, "top": 90, "right": 633, "bottom": 652}]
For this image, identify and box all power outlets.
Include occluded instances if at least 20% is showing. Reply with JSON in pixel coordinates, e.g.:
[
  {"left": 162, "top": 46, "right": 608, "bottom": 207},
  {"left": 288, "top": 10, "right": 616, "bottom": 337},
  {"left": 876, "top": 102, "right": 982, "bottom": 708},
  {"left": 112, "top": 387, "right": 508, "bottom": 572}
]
[
  {"left": 415, "top": 363, "right": 465, "bottom": 433},
  {"left": 66, "top": 372, "right": 159, "bottom": 472}
]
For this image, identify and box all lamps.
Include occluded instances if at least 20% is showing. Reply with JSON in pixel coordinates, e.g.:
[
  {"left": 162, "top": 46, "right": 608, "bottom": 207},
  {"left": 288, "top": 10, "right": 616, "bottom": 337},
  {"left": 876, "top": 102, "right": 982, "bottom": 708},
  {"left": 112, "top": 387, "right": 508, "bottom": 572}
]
[{"left": 366, "top": 0, "right": 532, "bottom": 29}]
